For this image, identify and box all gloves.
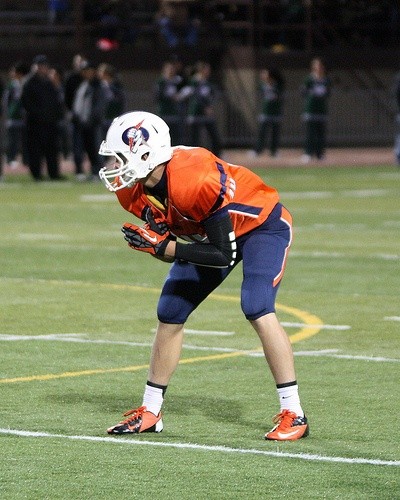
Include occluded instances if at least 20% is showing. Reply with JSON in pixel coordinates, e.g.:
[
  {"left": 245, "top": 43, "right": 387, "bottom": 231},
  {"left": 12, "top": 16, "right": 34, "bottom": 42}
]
[
  {"left": 122, "top": 224, "right": 170, "bottom": 256},
  {"left": 145, "top": 217, "right": 169, "bottom": 238}
]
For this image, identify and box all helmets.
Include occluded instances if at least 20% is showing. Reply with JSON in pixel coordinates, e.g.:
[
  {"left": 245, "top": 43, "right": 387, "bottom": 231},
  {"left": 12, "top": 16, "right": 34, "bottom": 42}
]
[{"left": 98, "top": 111, "right": 172, "bottom": 192}]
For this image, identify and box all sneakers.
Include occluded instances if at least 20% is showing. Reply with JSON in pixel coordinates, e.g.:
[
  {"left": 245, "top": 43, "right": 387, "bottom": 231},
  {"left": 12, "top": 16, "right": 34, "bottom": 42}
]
[
  {"left": 264, "top": 409, "right": 310, "bottom": 440},
  {"left": 107, "top": 407, "right": 164, "bottom": 435}
]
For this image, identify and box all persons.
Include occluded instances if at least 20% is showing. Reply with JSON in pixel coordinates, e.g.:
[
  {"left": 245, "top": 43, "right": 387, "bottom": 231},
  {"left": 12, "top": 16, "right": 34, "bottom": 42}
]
[
  {"left": 300, "top": 58, "right": 333, "bottom": 164},
  {"left": 184, "top": 61, "right": 224, "bottom": 162},
  {"left": 150, "top": 54, "right": 194, "bottom": 147},
  {"left": 246, "top": 65, "right": 285, "bottom": 159},
  {"left": 0, "top": 45, "right": 125, "bottom": 184},
  {"left": 93, "top": 110, "right": 309, "bottom": 442},
  {"left": 157, "top": 0, "right": 199, "bottom": 59}
]
